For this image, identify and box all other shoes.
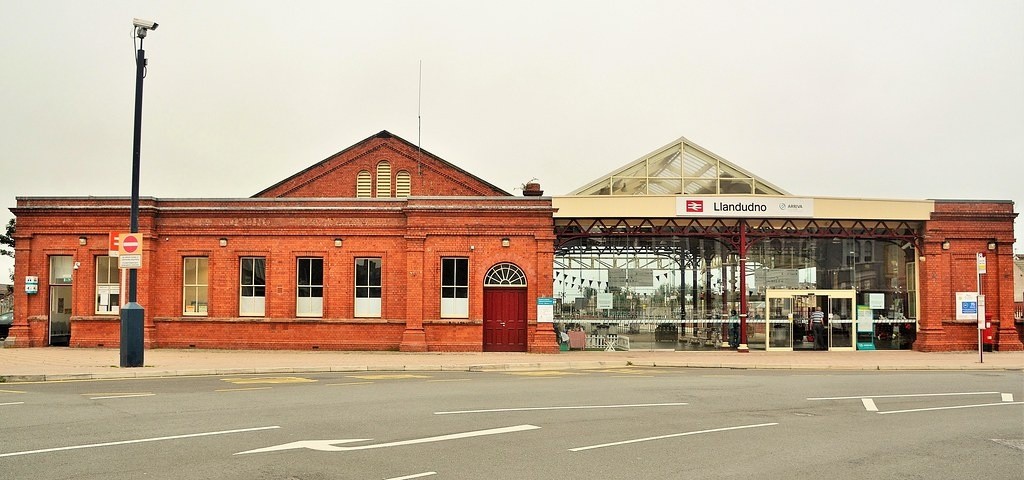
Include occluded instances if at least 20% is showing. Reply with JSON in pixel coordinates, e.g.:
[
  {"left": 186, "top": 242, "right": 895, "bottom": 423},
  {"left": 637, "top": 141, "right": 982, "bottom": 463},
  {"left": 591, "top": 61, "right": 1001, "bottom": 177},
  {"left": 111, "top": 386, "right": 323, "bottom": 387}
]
[
  {"left": 822, "top": 348, "right": 825, "bottom": 350},
  {"left": 731, "top": 344, "right": 738, "bottom": 348},
  {"left": 813, "top": 347, "right": 816, "bottom": 350}
]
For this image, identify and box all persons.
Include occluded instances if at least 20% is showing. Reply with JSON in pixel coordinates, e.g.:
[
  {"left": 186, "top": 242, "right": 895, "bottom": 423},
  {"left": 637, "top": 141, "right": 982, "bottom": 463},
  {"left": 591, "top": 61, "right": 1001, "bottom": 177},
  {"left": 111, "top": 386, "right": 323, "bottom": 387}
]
[
  {"left": 810, "top": 306, "right": 824, "bottom": 350},
  {"left": 831, "top": 309, "right": 843, "bottom": 346},
  {"left": 728, "top": 310, "right": 739, "bottom": 349}
]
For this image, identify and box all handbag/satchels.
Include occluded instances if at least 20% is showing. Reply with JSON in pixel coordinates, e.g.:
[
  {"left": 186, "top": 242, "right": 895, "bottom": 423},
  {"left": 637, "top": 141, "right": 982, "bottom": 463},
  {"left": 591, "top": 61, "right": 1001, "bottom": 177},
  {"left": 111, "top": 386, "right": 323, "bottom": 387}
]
[{"left": 733, "top": 323, "right": 739, "bottom": 328}]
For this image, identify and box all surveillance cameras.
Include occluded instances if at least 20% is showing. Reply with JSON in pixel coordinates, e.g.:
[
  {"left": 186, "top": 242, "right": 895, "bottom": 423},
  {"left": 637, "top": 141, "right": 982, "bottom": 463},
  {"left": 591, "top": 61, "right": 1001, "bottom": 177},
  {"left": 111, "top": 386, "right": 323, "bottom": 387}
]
[{"left": 132, "top": 18, "right": 159, "bottom": 31}]
